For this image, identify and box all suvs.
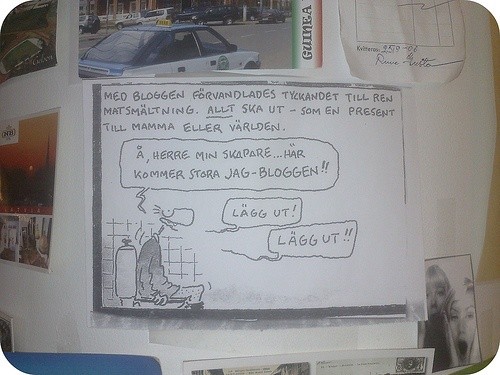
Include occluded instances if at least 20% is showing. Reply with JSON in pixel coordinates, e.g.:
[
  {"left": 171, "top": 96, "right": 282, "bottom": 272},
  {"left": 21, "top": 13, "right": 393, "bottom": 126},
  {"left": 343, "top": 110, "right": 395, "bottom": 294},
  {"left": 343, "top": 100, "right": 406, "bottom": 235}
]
[
  {"left": 192, "top": 3, "right": 239, "bottom": 26},
  {"left": 138, "top": 7, "right": 174, "bottom": 26},
  {"left": 114, "top": 11, "right": 144, "bottom": 30},
  {"left": 79, "top": 15, "right": 99, "bottom": 33}
]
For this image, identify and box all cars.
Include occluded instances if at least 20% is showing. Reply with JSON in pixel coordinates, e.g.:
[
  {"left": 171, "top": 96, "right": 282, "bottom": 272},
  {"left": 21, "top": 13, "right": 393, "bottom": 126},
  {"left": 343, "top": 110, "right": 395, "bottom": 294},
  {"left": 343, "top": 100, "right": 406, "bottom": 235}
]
[
  {"left": 79, "top": 19, "right": 260, "bottom": 78},
  {"left": 257, "top": 9, "right": 287, "bottom": 25}
]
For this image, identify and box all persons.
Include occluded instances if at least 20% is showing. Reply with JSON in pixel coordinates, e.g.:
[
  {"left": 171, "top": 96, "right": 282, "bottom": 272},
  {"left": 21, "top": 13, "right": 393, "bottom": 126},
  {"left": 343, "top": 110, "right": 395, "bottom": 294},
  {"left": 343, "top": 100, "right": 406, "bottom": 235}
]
[
  {"left": 417, "top": 264, "right": 454, "bottom": 371},
  {"left": 443, "top": 276, "right": 478, "bottom": 365}
]
